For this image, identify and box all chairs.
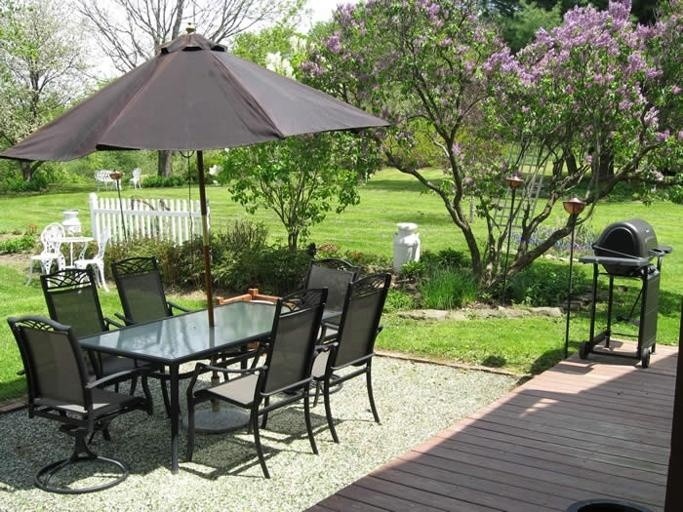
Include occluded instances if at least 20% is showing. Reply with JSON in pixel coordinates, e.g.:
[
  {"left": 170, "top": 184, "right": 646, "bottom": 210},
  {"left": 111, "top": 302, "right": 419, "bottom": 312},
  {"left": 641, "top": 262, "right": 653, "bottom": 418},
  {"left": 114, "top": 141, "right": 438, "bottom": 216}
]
[
  {"left": 73, "top": 229, "right": 111, "bottom": 295},
  {"left": 24, "top": 222, "right": 67, "bottom": 288},
  {"left": 259, "top": 273, "right": 391, "bottom": 443},
  {"left": 186, "top": 287, "right": 329, "bottom": 479},
  {"left": 110, "top": 257, "right": 195, "bottom": 401},
  {"left": 40, "top": 265, "right": 156, "bottom": 440},
  {"left": 129, "top": 167, "right": 142, "bottom": 191},
  {"left": 282, "top": 258, "right": 362, "bottom": 397},
  {"left": 7, "top": 315, "right": 131, "bottom": 495}
]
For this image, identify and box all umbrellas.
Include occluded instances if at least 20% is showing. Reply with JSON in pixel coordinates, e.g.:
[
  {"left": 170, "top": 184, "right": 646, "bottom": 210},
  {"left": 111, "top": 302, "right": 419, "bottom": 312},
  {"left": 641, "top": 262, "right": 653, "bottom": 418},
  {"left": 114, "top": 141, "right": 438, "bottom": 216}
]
[{"left": 1, "top": 23, "right": 391, "bottom": 329}]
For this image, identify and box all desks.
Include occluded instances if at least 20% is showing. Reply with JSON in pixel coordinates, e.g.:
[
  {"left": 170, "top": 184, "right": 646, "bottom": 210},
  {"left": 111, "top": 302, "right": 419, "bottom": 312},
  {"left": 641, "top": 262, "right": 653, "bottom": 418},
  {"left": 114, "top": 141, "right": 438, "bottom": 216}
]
[
  {"left": 50, "top": 236, "right": 94, "bottom": 270},
  {"left": 76, "top": 301, "right": 343, "bottom": 475},
  {"left": 94, "top": 170, "right": 124, "bottom": 192}
]
